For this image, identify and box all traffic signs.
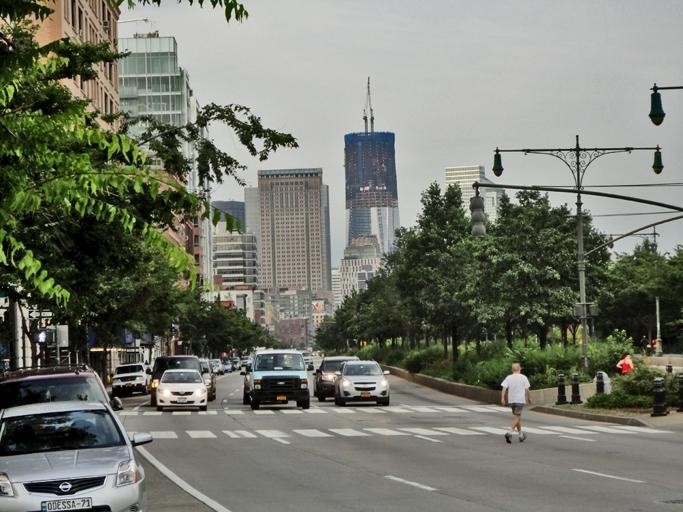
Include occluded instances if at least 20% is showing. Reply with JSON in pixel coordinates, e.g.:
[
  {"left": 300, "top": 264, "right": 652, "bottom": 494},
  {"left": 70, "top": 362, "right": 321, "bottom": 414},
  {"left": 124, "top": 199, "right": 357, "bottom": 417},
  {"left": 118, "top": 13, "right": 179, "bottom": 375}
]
[{"left": 27, "top": 309, "right": 54, "bottom": 320}]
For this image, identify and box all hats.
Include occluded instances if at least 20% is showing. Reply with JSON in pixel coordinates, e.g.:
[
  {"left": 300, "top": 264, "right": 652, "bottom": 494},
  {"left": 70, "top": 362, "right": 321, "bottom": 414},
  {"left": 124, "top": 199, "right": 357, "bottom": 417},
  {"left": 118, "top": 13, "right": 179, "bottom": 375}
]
[{"left": 512, "top": 363, "right": 521, "bottom": 369}]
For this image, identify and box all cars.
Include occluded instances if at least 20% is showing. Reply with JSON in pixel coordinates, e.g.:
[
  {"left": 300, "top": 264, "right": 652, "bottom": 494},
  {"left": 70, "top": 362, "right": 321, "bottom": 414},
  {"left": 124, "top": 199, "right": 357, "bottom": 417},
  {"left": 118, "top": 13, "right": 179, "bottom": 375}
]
[
  {"left": 144, "top": 349, "right": 392, "bottom": 411},
  {"left": 0, "top": 397, "right": 155, "bottom": 512}
]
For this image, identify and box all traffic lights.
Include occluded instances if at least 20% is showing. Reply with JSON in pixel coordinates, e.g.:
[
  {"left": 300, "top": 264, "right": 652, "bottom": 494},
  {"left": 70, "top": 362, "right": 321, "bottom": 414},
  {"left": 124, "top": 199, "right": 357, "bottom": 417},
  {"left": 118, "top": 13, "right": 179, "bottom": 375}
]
[{"left": 32, "top": 330, "right": 46, "bottom": 344}]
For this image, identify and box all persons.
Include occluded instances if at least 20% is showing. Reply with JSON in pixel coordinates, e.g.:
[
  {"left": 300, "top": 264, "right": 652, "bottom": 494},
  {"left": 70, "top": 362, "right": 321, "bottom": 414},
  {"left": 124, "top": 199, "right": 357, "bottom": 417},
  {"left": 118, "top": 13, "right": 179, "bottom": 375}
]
[
  {"left": 616, "top": 353, "right": 633, "bottom": 375},
  {"left": 500, "top": 363, "right": 532, "bottom": 443},
  {"left": 640, "top": 335, "right": 649, "bottom": 353},
  {"left": 651, "top": 335, "right": 657, "bottom": 353}
]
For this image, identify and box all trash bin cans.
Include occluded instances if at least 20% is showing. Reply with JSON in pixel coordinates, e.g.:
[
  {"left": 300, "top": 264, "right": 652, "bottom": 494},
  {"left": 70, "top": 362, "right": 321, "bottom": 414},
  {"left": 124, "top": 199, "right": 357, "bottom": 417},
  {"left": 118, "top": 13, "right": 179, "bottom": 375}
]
[{"left": 593, "top": 371, "right": 610, "bottom": 395}]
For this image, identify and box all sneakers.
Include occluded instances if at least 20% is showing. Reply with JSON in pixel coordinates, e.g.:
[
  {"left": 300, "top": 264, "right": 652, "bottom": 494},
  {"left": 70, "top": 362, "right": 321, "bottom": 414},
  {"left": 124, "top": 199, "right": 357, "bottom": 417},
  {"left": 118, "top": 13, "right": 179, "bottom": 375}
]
[{"left": 504, "top": 431, "right": 528, "bottom": 444}]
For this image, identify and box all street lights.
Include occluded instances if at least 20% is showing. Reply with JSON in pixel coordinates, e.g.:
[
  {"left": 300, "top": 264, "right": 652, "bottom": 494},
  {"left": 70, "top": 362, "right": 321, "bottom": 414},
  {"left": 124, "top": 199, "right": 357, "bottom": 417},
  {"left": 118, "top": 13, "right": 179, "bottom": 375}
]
[
  {"left": 491, "top": 133, "right": 665, "bottom": 376},
  {"left": 646, "top": 80, "right": 682, "bottom": 128}
]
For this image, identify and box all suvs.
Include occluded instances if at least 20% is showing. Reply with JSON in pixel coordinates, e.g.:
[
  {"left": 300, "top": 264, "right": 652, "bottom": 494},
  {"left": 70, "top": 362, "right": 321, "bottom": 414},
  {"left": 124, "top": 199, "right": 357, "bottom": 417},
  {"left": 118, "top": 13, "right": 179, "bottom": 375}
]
[
  {"left": 0, "top": 361, "right": 123, "bottom": 414},
  {"left": 108, "top": 361, "right": 154, "bottom": 394}
]
